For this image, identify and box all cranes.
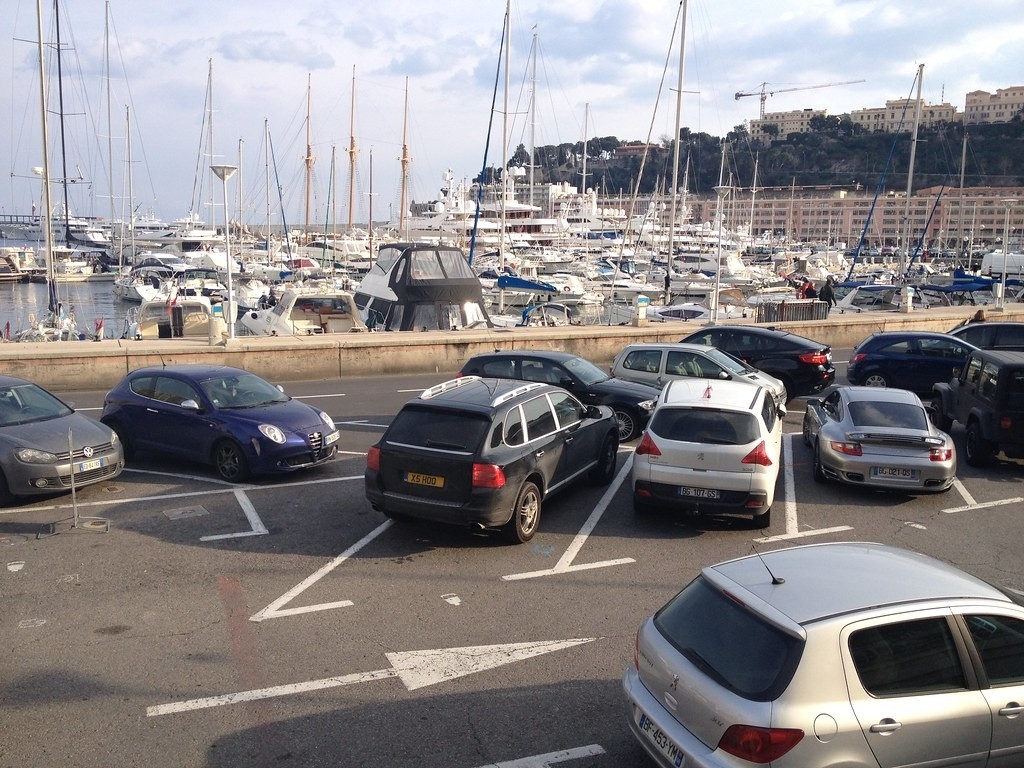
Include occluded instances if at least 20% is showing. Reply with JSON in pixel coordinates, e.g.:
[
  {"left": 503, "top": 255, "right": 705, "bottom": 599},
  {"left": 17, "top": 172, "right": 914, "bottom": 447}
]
[{"left": 735, "top": 79, "right": 866, "bottom": 149}]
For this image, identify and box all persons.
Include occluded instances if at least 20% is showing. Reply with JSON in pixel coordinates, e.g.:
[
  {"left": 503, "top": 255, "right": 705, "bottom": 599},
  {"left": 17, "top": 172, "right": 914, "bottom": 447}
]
[
  {"left": 260, "top": 287, "right": 276, "bottom": 309},
  {"left": 796, "top": 275, "right": 837, "bottom": 319}
]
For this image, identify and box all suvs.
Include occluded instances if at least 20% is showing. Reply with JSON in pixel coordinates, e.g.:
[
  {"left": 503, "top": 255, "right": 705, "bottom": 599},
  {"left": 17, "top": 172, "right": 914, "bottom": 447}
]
[
  {"left": 630, "top": 377, "right": 786, "bottom": 529},
  {"left": 931, "top": 348, "right": 1024, "bottom": 471},
  {"left": 363, "top": 376, "right": 620, "bottom": 545}
]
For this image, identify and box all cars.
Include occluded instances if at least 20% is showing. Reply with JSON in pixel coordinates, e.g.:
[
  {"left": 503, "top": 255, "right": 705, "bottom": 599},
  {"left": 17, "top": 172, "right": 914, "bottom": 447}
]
[
  {"left": 621, "top": 538, "right": 1024, "bottom": 768},
  {"left": 98, "top": 363, "right": 341, "bottom": 482},
  {"left": 454, "top": 350, "right": 663, "bottom": 441},
  {"left": 0, "top": 373, "right": 125, "bottom": 506},
  {"left": 846, "top": 245, "right": 1023, "bottom": 260},
  {"left": 846, "top": 331, "right": 983, "bottom": 397},
  {"left": 802, "top": 385, "right": 958, "bottom": 494},
  {"left": 611, "top": 340, "right": 787, "bottom": 410},
  {"left": 944, "top": 318, "right": 1024, "bottom": 348},
  {"left": 678, "top": 324, "right": 834, "bottom": 399}
]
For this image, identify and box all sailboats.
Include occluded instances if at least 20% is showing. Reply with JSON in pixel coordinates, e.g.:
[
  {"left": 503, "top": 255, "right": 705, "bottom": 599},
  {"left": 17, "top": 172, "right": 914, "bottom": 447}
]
[{"left": 1, "top": 3, "right": 1024, "bottom": 338}]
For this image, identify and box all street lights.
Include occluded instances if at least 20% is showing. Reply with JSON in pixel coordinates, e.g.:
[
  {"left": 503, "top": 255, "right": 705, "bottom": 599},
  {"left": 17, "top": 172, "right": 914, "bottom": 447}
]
[{"left": 208, "top": 163, "right": 240, "bottom": 334}]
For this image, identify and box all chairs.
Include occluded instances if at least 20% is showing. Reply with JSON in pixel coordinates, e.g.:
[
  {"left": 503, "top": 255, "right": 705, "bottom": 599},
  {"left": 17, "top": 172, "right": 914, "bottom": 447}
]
[
  {"left": 186, "top": 278, "right": 217, "bottom": 289},
  {"left": 183, "top": 313, "right": 209, "bottom": 336},
  {"left": 292, "top": 306, "right": 353, "bottom": 335},
  {"left": 141, "top": 319, "right": 159, "bottom": 339}
]
